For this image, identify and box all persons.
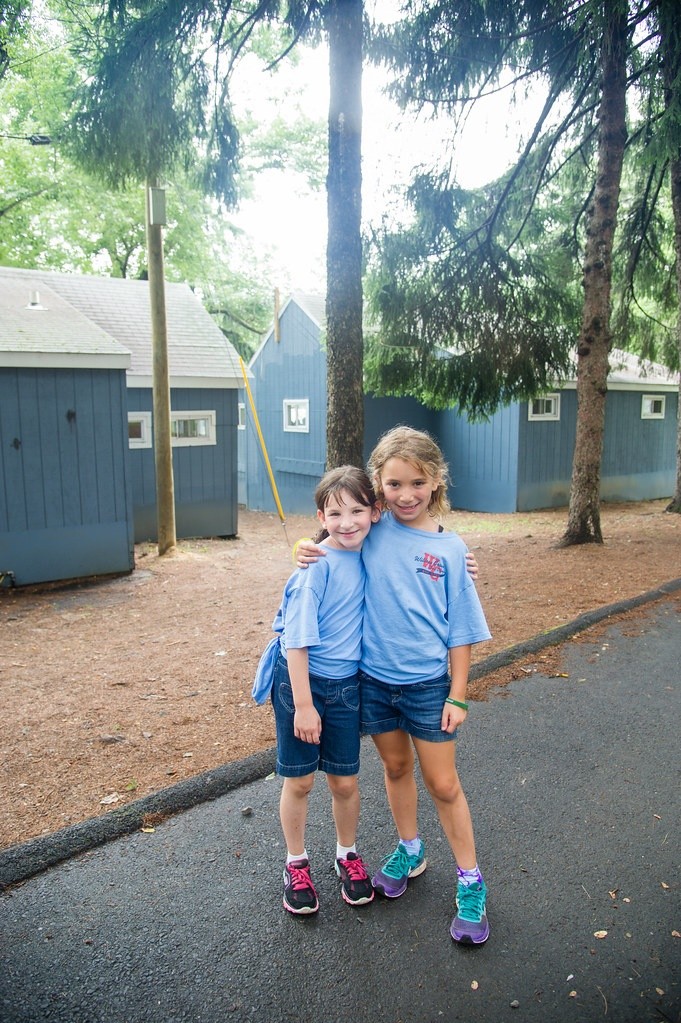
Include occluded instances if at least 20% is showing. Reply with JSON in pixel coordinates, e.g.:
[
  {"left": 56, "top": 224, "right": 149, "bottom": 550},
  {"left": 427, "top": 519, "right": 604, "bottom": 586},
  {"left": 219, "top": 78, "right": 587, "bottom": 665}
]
[
  {"left": 292, "top": 428, "right": 492, "bottom": 944},
  {"left": 252, "top": 465, "right": 478, "bottom": 914}
]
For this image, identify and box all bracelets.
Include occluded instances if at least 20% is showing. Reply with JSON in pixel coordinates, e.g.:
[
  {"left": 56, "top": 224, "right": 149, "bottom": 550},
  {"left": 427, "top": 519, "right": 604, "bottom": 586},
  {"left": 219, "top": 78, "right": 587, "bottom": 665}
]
[
  {"left": 293, "top": 538, "right": 311, "bottom": 560},
  {"left": 445, "top": 698, "right": 468, "bottom": 710}
]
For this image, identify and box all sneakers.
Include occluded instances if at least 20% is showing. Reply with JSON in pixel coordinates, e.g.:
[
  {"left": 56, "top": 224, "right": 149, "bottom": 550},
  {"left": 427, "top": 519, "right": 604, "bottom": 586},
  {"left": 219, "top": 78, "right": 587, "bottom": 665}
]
[
  {"left": 371, "top": 840, "right": 427, "bottom": 898},
  {"left": 450, "top": 878, "right": 490, "bottom": 944},
  {"left": 334, "top": 851, "right": 374, "bottom": 905},
  {"left": 282, "top": 859, "right": 319, "bottom": 914}
]
